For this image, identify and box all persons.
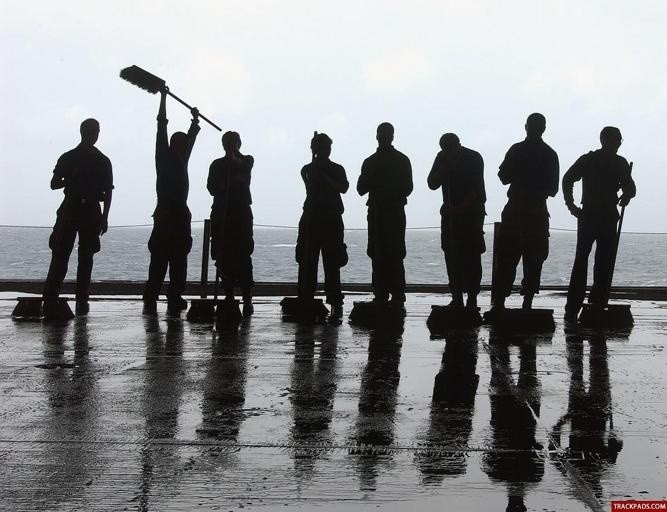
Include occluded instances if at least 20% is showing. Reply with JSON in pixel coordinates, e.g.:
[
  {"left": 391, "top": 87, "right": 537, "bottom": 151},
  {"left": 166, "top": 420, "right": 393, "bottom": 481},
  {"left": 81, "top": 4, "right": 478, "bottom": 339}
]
[
  {"left": 206, "top": 131, "right": 254, "bottom": 316},
  {"left": 428, "top": 133, "right": 487, "bottom": 306},
  {"left": 43, "top": 117, "right": 114, "bottom": 315},
  {"left": 352, "top": 310, "right": 407, "bottom": 500},
  {"left": 357, "top": 122, "right": 413, "bottom": 307},
  {"left": 490, "top": 112, "right": 559, "bottom": 310},
  {"left": 142, "top": 85, "right": 201, "bottom": 309},
  {"left": 485, "top": 315, "right": 549, "bottom": 511},
  {"left": 549, "top": 320, "right": 633, "bottom": 510},
  {"left": 562, "top": 126, "right": 636, "bottom": 325},
  {"left": 427, "top": 305, "right": 487, "bottom": 487},
  {"left": 296, "top": 133, "right": 349, "bottom": 317}
]
